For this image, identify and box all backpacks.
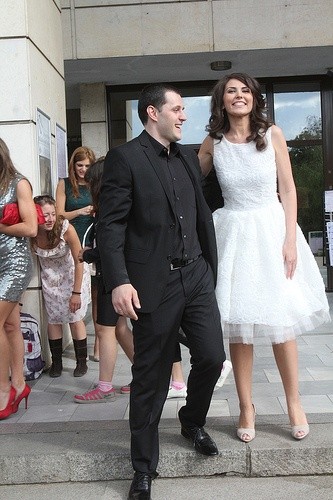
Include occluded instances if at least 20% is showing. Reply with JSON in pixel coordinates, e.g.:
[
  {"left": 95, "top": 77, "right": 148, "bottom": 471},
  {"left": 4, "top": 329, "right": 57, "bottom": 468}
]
[{"left": 9, "top": 303, "right": 46, "bottom": 381}]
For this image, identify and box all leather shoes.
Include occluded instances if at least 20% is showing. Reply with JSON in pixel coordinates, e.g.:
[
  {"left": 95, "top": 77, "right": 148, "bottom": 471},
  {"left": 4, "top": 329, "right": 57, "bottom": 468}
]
[
  {"left": 181, "top": 424, "right": 219, "bottom": 455},
  {"left": 128, "top": 470, "right": 159, "bottom": 500}
]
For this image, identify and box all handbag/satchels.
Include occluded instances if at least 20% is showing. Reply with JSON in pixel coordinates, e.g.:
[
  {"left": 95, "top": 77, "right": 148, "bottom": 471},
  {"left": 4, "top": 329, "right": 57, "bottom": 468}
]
[
  {"left": 81, "top": 223, "right": 97, "bottom": 276},
  {"left": 0, "top": 203, "right": 46, "bottom": 225}
]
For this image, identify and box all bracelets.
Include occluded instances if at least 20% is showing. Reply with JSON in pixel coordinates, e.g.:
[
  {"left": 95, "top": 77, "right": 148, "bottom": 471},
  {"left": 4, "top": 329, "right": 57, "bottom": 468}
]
[{"left": 71, "top": 290, "right": 81, "bottom": 295}]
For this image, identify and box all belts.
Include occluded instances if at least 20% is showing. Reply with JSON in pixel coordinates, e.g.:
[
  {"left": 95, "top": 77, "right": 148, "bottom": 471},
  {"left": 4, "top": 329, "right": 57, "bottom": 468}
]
[{"left": 170, "top": 252, "right": 199, "bottom": 270}]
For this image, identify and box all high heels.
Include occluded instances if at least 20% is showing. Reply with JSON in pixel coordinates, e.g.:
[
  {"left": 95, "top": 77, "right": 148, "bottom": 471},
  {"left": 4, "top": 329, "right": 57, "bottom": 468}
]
[
  {"left": 290, "top": 413, "right": 310, "bottom": 440},
  {"left": 11, "top": 383, "right": 31, "bottom": 413},
  {"left": 237, "top": 402, "right": 256, "bottom": 443},
  {"left": 0, "top": 384, "right": 16, "bottom": 419}
]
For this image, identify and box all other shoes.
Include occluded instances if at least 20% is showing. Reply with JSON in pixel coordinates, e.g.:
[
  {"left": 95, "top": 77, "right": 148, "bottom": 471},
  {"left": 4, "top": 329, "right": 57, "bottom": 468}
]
[
  {"left": 213, "top": 360, "right": 233, "bottom": 392},
  {"left": 121, "top": 383, "right": 131, "bottom": 394},
  {"left": 166, "top": 385, "right": 188, "bottom": 398},
  {"left": 74, "top": 385, "right": 116, "bottom": 403},
  {"left": 93, "top": 355, "right": 100, "bottom": 361}
]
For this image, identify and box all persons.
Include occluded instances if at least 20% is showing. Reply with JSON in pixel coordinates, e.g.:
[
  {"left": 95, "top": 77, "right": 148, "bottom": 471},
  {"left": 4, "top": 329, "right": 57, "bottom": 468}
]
[
  {"left": 194, "top": 72, "right": 330, "bottom": 444},
  {"left": 96, "top": 85, "right": 225, "bottom": 500},
  {"left": 0, "top": 137, "right": 232, "bottom": 420}
]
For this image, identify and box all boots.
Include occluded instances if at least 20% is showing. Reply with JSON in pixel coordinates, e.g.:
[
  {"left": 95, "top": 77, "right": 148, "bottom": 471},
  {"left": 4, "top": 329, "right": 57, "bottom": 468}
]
[
  {"left": 48, "top": 338, "right": 63, "bottom": 377},
  {"left": 73, "top": 337, "right": 88, "bottom": 377}
]
